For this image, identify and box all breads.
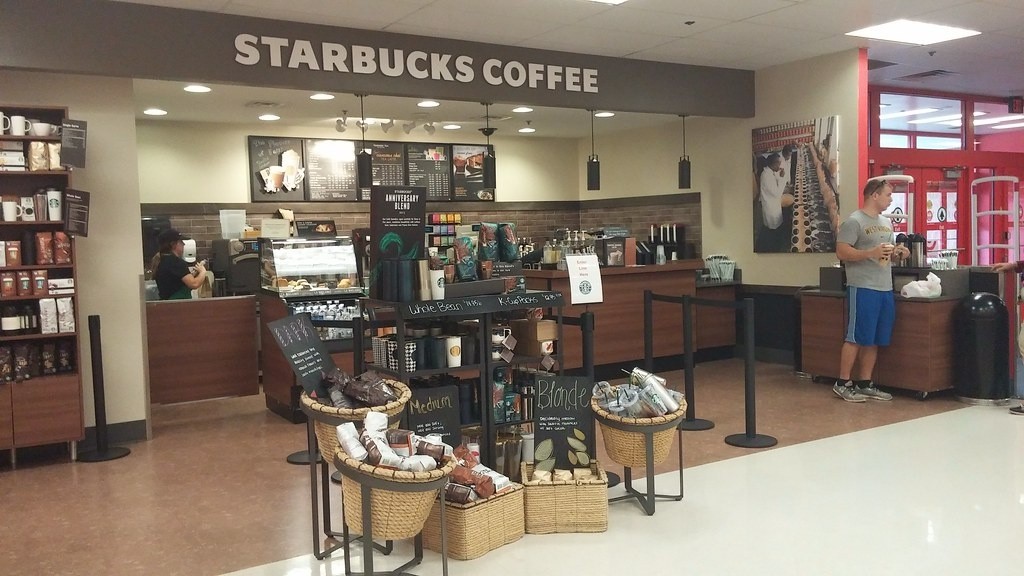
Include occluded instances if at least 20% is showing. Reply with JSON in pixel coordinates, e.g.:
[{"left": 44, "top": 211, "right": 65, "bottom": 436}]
[{"left": 270, "top": 275, "right": 352, "bottom": 288}]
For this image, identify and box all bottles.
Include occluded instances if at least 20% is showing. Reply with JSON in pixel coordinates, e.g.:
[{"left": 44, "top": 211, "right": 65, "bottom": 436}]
[
  {"left": 543, "top": 238, "right": 558, "bottom": 264},
  {"left": 517, "top": 237, "right": 534, "bottom": 255}
]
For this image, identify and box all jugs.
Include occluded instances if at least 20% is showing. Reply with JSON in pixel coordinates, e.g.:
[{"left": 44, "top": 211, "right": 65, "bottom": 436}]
[{"left": 896, "top": 233, "right": 925, "bottom": 268}]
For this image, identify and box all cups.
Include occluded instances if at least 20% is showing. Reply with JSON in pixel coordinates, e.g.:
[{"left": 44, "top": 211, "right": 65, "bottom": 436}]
[
  {"left": 930, "top": 250, "right": 958, "bottom": 270},
  {"left": 410, "top": 375, "right": 472, "bottom": 424},
  {"left": 3, "top": 201, "right": 23, "bottom": 222},
  {"left": 592, "top": 367, "right": 682, "bottom": 419},
  {"left": 0, "top": 111, "right": 62, "bottom": 137},
  {"left": 382, "top": 259, "right": 445, "bottom": 300},
  {"left": 513, "top": 380, "right": 533, "bottom": 420},
  {"left": 879, "top": 243, "right": 893, "bottom": 266},
  {"left": 268, "top": 166, "right": 285, "bottom": 188},
  {"left": 34, "top": 190, "right": 63, "bottom": 221},
  {"left": 282, "top": 153, "right": 299, "bottom": 184},
  {"left": 370, "top": 323, "right": 478, "bottom": 373}
]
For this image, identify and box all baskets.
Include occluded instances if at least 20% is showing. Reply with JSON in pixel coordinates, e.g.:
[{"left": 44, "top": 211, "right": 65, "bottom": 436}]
[
  {"left": 334, "top": 442, "right": 458, "bottom": 540},
  {"left": 520, "top": 459, "right": 609, "bottom": 533},
  {"left": 422, "top": 483, "right": 526, "bottom": 561},
  {"left": 302, "top": 380, "right": 412, "bottom": 463},
  {"left": 592, "top": 383, "right": 687, "bottom": 467}
]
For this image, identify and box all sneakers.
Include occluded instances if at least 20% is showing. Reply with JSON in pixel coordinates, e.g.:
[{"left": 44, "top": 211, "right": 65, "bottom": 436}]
[
  {"left": 832, "top": 381, "right": 866, "bottom": 402},
  {"left": 1010, "top": 405, "right": 1024, "bottom": 415},
  {"left": 854, "top": 381, "right": 893, "bottom": 400}
]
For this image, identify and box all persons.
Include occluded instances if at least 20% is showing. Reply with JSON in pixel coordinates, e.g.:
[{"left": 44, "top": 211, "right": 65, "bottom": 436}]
[
  {"left": 991, "top": 260, "right": 1024, "bottom": 415},
  {"left": 753, "top": 134, "right": 839, "bottom": 230},
  {"left": 834, "top": 178, "right": 913, "bottom": 402},
  {"left": 150, "top": 229, "right": 207, "bottom": 300}
]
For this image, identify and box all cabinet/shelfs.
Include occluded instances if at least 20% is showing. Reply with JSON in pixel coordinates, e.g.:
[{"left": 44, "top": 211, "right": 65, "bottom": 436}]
[
  {"left": 357, "top": 285, "right": 566, "bottom": 487},
  {"left": 0, "top": 103, "right": 86, "bottom": 467}
]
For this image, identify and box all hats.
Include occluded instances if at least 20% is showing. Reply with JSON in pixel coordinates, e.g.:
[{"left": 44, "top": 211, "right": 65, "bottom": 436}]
[{"left": 159, "top": 229, "right": 191, "bottom": 242}]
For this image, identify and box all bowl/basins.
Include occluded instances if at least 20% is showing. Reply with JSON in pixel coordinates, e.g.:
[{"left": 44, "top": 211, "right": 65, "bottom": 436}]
[{"left": 465, "top": 154, "right": 483, "bottom": 170}]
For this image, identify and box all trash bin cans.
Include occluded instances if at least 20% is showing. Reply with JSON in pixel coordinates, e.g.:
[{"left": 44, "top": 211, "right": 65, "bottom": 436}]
[{"left": 950, "top": 291, "right": 1011, "bottom": 406}]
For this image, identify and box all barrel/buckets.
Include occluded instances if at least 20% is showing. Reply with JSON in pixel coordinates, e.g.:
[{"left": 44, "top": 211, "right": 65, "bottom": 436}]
[{"left": 520, "top": 433, "right": 534, "bottom": 462}]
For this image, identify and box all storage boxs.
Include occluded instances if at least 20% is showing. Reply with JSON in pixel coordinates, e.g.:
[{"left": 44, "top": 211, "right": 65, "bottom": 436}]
[
  {"left": 0, "top": 241, "right": 7, "bottom": 267},
  {"left": 500, "top": 275, "right": 526, "bottom": 294},
  {"left": 0, "top": 156, "right": 25, "bottom": 171},
  {"left": 0, "top": 272, "right": 17, "bottom": 297},
  {"left": 445, "top": 277, "right": 505, "bottom": 298},
  {"left": 16, "top": 270, "right": 33, "bottom": 296},
  {"left": 0, "top": 141, "right": 24, "bottom": 156},
  {"left": 509, "top": 319, "right": 559, "bottom": 341},
  {"left": 491, "top": 260, "right": 522, "bottom": 277},
  {"left": 31, "top": 270, "right": 48, "bottom": 295},
  {"left": 5, "top": 241, "right": 22, "bottom": 266}
]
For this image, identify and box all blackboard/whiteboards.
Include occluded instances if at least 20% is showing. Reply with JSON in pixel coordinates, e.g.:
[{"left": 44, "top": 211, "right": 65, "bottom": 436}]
[
  {"left": 406, "top": 384, "right": 462, "bottom": 451},
  {"left": 534, "top": 375, "right": 596, "bottom": 471},
  {"left": 266, "top": 312, "right": 336, "bottom": 397}
]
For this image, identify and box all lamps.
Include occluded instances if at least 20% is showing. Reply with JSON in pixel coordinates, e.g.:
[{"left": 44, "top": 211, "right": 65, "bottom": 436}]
[
  {"left": 477, "top": 102, "right": 497, "bottom": 189},
  {"left": 402, "top": 120, "right": 419, "bottom": 134},
  {"left": 678, "top": 114, "right": 691, "bottom": 189},
  {"left": 335, "top": 110, "right": 348, "bottom": 132},
  {"left": 353, "top": 92, "right": 374, "bottom": 189},
  {"left": 356, "top": 118, "right": 368, "bottom": 132},
  {"left": 586, "top": 108, "right": 600, "bottom": 191},
  {"left": 423, "top": 121, "right": 436, "bottom": 135},
  {"left": 380, "top": 118, "right": 394, "bottom": 134}
]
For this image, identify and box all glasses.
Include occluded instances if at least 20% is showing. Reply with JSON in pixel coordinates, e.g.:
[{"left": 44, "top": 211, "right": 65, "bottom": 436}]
[{"left": 871, "top": 179, "right": 887, "bottom": 196}]
